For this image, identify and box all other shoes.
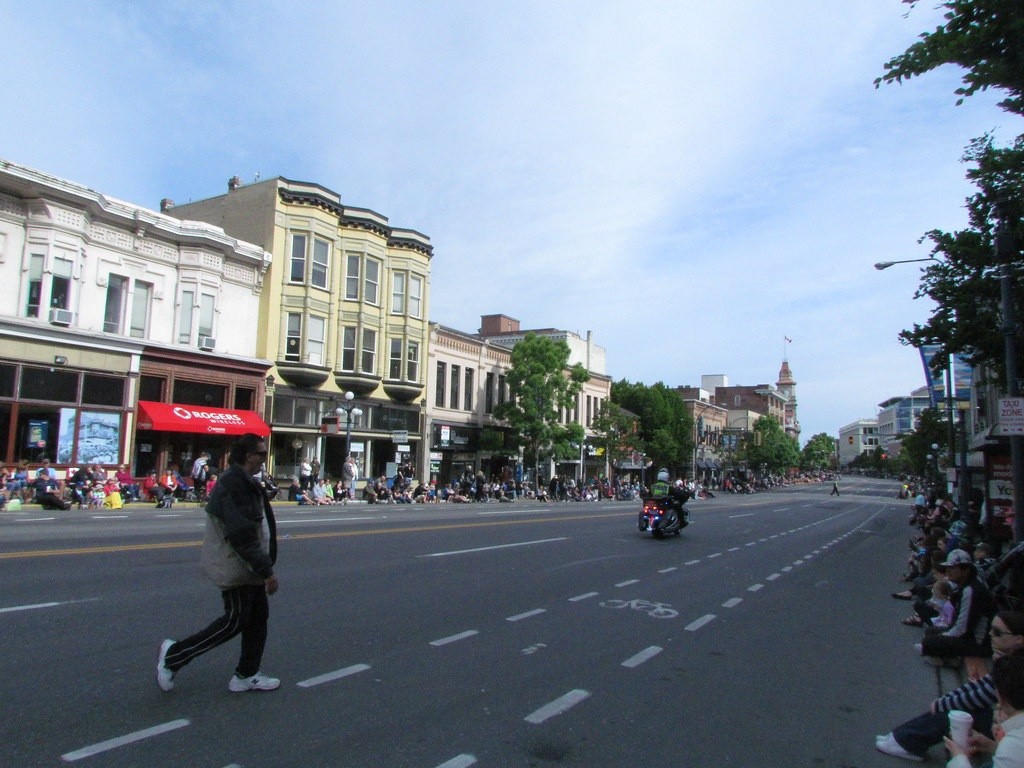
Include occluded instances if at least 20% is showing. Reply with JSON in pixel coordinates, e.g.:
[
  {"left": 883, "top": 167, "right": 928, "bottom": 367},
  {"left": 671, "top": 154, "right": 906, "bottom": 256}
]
[
  {"left": 891, "top": 593, "right": 911, "bottom": 600},
  {"left": 923, "top": 655, "right": 962, "bottom": 668}
]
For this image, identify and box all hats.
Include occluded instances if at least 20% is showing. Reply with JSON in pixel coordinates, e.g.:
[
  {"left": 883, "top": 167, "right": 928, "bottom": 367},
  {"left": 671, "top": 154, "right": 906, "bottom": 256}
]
[
  {"left": 973, "top": 542, "right": 992, "bottom": 552},
  {"left": 96, "top": 485, "right": 104, "bottom": 491},
  {"left": 39, "top": 469, "right": 49, "bottom": 475},
  {"left": 554, "top": 475, "right": 558, "bottom": 479},
  {"left": 949, "top": 521, "right": 968, "bottom": 537},
  {"left": 43, "top": 459, "right": 49, "bottom": 462},
  {"left": 941, "top": 548, "right": 972, "bottom": 566}
]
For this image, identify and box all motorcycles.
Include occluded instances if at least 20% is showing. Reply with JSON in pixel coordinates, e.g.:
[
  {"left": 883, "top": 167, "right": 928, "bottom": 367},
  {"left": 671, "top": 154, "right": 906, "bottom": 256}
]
[{"left": 637, "top": 487, "right": 693, "bottom": 539}]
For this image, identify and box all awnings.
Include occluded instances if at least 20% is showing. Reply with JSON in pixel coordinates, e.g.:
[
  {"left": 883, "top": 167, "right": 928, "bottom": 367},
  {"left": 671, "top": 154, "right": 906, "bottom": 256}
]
[{"left": 137, "top": 399, "right": 269, "bottom": 438}]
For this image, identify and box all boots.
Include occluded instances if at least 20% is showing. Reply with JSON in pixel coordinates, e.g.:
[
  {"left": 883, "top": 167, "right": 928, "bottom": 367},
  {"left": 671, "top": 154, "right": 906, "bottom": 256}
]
[{"left": 677, "top": 509, "right": 688, "bottom": 528}]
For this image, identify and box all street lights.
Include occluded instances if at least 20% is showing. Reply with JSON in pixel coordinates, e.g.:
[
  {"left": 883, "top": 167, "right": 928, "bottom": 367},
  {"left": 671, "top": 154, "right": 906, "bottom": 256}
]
[
  {"left": 874, "top": 257, "right": 959, "bottom": 500},
  {"left": 724, "top": 416, "right": 751, "bottom": 493},
  {"left": 694, "top": 402, "right": 727, "bottom": 493},
  {"left": 927, "top": 440, "right": 939, "bottom": 500},
  {"left": 334, "top": 390, "right": 364, "bottom": 494},
  {"left": 573, "top": 433, "right": 594, "bottom": 499}
]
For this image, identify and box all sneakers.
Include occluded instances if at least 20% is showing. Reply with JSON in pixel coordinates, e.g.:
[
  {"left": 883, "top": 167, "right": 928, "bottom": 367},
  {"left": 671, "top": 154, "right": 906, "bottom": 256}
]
[
  {"left": 229, "top": 672, "right": 281, "bottom": 691},
  {"left": 876, "top": 732, "right": 922, "bottom": 761},
  {"left": 901, "top": 615, "right": 923, "bottom": 626},
  {"left": 157, "top": 639, "right": 175, "bottom": 692}
]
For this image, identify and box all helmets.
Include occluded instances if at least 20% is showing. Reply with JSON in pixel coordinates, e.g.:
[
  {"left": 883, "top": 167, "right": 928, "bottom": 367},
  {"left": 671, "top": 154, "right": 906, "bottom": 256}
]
[{"left": 658, "top": 471, "right": 669, "bottom": 482}]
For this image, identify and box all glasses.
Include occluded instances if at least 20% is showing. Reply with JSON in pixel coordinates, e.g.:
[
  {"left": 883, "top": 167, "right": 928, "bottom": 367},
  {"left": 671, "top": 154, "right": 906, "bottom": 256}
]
[
  {"left": 256, "top": 452, "right": 267, "bottom": 457},
  {"left": 992, "top": 628, "right": 1012, "bottom": 636}
]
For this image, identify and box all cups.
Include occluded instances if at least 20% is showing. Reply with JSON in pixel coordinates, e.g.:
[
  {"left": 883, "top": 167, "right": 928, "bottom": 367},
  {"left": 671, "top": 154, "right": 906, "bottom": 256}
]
[{"left": 948, "top": 710, "right": 974, "bottom": 750}]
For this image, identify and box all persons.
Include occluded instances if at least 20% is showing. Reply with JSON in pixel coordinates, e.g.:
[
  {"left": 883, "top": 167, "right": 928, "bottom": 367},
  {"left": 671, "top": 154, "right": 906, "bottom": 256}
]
[
  {"left": 648, "top": 468, "right": 689, "bottom": 528},
  {"left": 875, "top": 472, "right": 1024, "bottom": 768},
  {"left": 0, "top": 457, "right": 652, "bottom": 510},
  {"left": 156, "top": 433, "right": 282, "bottom": 693},
  {"left": 675, "top": 471, "right": 843, "bottom": 502}
]
